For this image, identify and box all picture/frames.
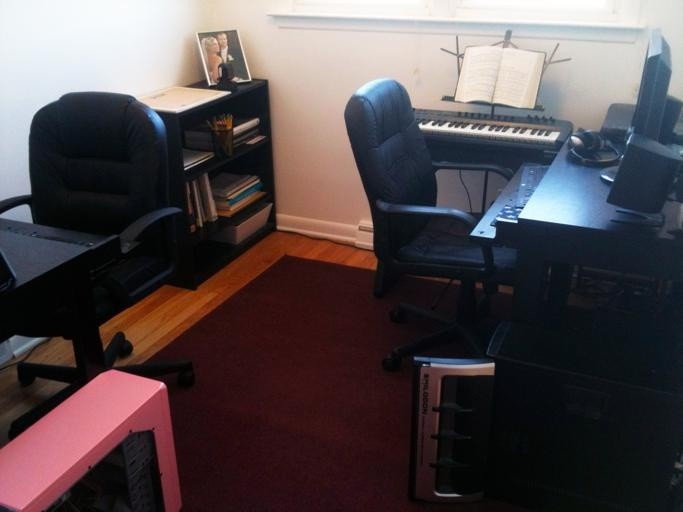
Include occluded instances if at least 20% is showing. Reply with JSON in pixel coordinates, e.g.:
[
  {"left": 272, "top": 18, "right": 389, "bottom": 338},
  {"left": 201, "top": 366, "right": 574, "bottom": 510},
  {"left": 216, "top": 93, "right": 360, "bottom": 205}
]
[{"left": 195, "top": 29, "right": 255, "bottom": 86}]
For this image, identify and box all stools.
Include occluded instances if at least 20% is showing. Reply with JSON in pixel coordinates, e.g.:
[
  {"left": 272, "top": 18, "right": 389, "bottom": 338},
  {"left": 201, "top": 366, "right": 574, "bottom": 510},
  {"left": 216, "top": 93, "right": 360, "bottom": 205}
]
[{"left": 1, "top": 369, "right": 182, "bottom": 511}]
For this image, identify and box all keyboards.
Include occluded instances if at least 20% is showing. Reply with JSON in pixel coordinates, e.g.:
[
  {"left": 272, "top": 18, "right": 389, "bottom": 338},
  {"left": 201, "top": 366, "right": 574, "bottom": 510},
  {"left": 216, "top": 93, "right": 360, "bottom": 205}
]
[{"left": 515, "top": 166, "right": 550, "bottom": 208}]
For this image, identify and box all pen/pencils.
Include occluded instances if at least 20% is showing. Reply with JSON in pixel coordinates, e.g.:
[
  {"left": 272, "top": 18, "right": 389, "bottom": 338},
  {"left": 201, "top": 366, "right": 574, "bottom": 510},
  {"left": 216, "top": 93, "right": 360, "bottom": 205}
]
[{"left": 207, "top": 113, "right": 233, "bottom": 130}]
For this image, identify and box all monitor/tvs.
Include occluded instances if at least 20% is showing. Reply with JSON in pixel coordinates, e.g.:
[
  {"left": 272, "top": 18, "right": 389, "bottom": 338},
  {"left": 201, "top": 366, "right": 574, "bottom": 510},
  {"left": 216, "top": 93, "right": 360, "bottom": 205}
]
[{"left": 599, "top": 28, "right": 672, "bottom": 185}]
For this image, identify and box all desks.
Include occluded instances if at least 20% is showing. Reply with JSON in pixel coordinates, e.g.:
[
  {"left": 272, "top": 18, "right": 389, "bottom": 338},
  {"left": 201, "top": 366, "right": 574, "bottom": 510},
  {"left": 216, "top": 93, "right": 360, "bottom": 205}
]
[
  {"left": 1, "top": 214, "right": 125, "bottom": 510},
  {"left": 469, "top": 128, "right": 683, "bottom": 396}
]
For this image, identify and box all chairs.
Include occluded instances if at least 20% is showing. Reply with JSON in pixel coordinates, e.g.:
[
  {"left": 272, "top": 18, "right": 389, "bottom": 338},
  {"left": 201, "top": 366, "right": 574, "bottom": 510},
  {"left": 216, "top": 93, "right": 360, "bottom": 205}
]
[
  {"left": 1, "top": 91, "right": 198, "bottom": 446},
  {"left": 344, "top": 76, "right": 533, "bottom": 371}
]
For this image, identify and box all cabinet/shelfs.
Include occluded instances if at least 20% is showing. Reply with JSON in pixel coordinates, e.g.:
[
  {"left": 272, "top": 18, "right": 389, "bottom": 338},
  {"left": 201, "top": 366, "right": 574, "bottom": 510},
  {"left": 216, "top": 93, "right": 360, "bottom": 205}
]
[{"left": 133, "top": 76, "right": 277, "bottom": 291}]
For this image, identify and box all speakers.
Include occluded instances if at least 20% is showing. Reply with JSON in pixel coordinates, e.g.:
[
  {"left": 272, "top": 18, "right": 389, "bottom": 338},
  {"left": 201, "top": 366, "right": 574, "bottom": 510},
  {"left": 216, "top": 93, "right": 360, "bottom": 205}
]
[
  {"left": 606, "top": 134, "right": 683, "bottom": 227},
  {"left": 659, "top": 96, "right": 683, "bottom": 143}
]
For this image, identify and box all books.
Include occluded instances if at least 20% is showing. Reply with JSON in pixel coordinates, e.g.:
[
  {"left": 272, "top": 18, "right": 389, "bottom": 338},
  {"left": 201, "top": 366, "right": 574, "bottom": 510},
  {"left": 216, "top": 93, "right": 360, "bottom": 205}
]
[
  {"left": 453, "top": 45, "right": 546, "bottom": 110},
  {"left": 183, "top": 148, "right": 267, "bottom": 232}
]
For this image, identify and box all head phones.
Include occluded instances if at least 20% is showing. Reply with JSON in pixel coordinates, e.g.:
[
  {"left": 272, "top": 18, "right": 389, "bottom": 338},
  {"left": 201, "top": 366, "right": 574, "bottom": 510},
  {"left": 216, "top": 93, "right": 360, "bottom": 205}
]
[{"left": 569, "top": 130, "right": 622, "bottom": 167}]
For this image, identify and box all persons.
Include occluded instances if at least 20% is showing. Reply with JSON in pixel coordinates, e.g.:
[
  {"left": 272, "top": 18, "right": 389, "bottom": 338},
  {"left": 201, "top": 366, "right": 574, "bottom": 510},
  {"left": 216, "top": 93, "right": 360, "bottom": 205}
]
[{"left": 201, "top": 33, "right": 247, "bottom": 84}]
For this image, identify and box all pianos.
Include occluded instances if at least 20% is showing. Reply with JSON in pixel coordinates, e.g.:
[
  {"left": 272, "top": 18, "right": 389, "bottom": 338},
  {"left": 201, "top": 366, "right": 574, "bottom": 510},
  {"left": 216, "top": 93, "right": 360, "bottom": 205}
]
[{"left": 414, "top": 109, "right": 573, "bottom": 152}]
